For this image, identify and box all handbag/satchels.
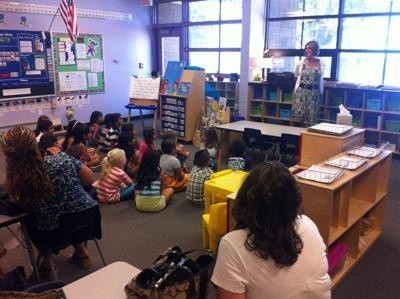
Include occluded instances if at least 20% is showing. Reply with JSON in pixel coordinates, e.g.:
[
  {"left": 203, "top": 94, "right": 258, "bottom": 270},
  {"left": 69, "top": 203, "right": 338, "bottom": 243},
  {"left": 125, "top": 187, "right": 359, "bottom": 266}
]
[{"left": 124, "top": 246, "right": 216, "bottom": 299}]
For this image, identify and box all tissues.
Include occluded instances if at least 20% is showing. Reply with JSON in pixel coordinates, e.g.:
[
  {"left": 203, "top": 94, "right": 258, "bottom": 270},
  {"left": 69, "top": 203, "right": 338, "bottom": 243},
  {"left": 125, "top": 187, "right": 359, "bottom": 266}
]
[{"left": 336, "top": 104, "right": 352, "bottom": 126}]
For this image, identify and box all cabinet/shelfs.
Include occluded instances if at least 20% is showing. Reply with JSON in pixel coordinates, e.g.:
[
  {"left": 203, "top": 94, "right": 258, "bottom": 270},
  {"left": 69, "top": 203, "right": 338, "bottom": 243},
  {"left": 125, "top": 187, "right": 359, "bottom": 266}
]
[
  {"left": 206, "top": 80, "right": 238, "bottom": 117},
  {"left": 247, "top": 81, "right": 400, "bottom": 156},
  {"left": 159, "top": 69, "right": 205, "bottom": 142},
  {"left": 292, "top": 145, "right": 392, "bottom": 289}
]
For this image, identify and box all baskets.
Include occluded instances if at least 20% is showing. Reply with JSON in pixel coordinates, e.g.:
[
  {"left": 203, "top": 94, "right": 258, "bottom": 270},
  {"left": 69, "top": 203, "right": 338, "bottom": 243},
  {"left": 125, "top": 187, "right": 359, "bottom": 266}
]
[
  {"left": 321, "top": 151, "right": 368, "bottom": 170},
  {"left": 345, "top": 135, "right": 390, "bottom": 158},
  {"left": 296, "top": 159, "right": 347, "bottom": 184}
]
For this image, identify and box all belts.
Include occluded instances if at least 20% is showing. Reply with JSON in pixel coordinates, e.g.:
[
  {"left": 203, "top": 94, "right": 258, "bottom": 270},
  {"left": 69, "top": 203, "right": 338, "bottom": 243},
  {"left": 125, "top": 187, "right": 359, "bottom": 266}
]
[{"left": 300, "top": 84, "right": 318, "bottom": 90}]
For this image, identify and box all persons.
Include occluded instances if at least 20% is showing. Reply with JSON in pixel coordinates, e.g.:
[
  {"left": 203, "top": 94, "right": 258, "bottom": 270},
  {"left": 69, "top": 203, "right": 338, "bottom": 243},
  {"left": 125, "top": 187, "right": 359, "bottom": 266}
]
[
  {"left": 210, "top": 161, "right": 331, "bottom": 299},
  {"left": 291, "top": 41, "right": 324, "bottom": 125},
  {"left": 0, "top": 112, "right": 253, "bottom": 270}
]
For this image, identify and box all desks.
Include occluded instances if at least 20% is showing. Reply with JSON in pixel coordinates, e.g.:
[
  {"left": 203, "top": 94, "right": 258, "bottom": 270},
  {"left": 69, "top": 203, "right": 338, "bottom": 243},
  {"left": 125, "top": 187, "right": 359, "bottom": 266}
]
[
  {"left": 215, "top": 120, "right": 307, "bottom": 166},
  {"left": 58, "top": 261, "right": 143, "bottom": 299},
  {"left": 299, "top": 124, "right": 366, "bottom": 168},
  {"left": 124, "top": 104, "right": 158, "bottom": 129},
  {"left": 203, "top": 171, "right": 250, "bottom": 214},
  {"left": 0, "top": 210, "right": 27, "bottom": 249}
]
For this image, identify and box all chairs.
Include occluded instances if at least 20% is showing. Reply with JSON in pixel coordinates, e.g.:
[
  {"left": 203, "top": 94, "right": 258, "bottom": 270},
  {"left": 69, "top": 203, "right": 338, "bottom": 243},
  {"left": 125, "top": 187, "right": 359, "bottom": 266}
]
[
  {"left": 26, "top": 205, "right": 106, "bottom": 280},
  {"left": 210, "top": 168, "right": 232, "bottom": 203},
  {"left": 201, "top": 202, "right": 227, "bottom": 251},
  {"left": 243, "top": 128, "right": 273, "bottom": 155},
  {"left": 280, "top": 133, "right": 300, "bottom": 161}
]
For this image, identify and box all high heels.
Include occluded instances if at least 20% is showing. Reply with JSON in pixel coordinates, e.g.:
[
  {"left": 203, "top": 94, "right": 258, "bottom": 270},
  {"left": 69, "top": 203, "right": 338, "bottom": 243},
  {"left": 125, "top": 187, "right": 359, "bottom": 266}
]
[
  {"left": 39, "top": 264, "right": 57, "bottom": 280},
  {"left": 68, "top": 252, "right": 93, "bottom": 268}
]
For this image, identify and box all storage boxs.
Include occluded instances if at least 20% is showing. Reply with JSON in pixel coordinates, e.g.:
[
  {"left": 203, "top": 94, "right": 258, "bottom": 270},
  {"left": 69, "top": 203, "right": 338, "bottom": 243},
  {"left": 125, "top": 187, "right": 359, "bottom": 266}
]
[{"left": 252, "top": 92, "right": 400, "bottom": 132}]
